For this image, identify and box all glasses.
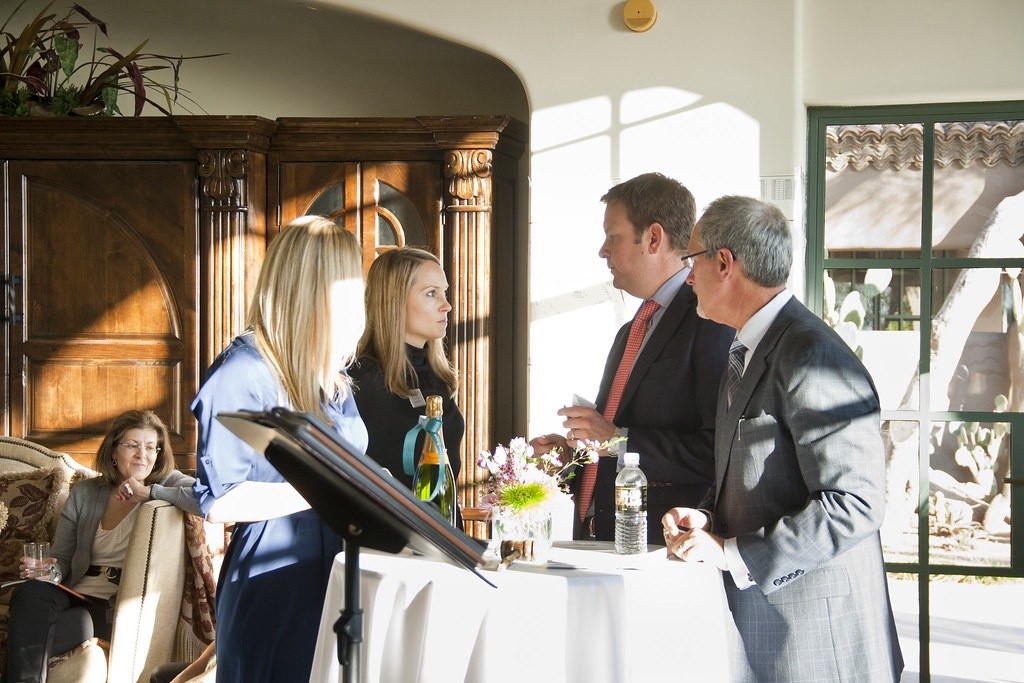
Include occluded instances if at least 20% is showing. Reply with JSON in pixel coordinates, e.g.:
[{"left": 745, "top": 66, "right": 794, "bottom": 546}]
[
  {"left": 678, "top": 246, "right": 737, "bottom": 270},
  {"left": 115, "top": 441, "right": 161, "bottom": 456}
]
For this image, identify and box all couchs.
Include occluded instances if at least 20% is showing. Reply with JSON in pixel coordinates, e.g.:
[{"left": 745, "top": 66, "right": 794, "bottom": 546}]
[{"left": 0, "top": 436, "right": 193, "bottom": 683}]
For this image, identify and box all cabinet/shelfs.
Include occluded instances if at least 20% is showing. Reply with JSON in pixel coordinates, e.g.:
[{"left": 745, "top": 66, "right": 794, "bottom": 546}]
[
  {"left": 265, "top": 114, "right": 530, "bottom": 545},
  {"left": 0, "top": 114, "right": 272, "bottom": 542}
]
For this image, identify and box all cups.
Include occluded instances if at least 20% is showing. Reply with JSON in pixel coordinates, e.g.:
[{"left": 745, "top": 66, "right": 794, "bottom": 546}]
[{"left": 24, "top": 542, "right": 51, "bottom": 578}]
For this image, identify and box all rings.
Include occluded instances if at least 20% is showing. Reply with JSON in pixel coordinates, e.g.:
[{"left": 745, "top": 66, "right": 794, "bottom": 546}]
[
  {"left": 571, "top": 429, "right": 575, "bottom": 440},
  {"left": 663, "top": 527, "right": 669, "bottom": 539}
]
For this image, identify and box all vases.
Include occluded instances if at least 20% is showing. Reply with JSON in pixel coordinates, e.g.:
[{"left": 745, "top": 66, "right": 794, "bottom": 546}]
[{"left": 500, "top": 538, "right": 548, "bottom": 569}]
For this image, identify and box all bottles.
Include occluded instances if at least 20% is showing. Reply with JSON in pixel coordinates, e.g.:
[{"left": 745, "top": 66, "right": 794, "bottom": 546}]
[
  {"left": 413, "top": 396, "right": 457, "bottom": 527},
  {"left": 615, "top": 452, "right": 648, "bottom": 554}
]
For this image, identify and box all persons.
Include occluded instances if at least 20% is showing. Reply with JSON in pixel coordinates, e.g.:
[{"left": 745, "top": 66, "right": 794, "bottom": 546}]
[
  {"left": 6, "top": 409, "right": 201, "bottom": 683},
  {"left": 529, "top": 172, "right": 737, "bottom": 546},
  {"left": 346, "top": 248, "right": 464, "bottom": 554},
  {"left": 662, "top": 196, "right": 905, "bottom": 683},
  {"left": 191, "top": 215, "right": 369, "bottom": 683}
]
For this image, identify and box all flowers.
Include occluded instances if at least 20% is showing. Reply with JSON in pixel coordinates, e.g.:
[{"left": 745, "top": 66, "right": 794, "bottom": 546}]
[{"left": 474, "top": 432, "right": 629, "bottom": 541}]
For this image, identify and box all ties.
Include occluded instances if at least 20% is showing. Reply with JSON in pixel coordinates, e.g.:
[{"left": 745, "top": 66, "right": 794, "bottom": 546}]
[
  {"left": 575, "top": 300, "right": 660, "bottom": 524},
  {"left": 726, "top": 337, "right": 747, "bottom": 406}
]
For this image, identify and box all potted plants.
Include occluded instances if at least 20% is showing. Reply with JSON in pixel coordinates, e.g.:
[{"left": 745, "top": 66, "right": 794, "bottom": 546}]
[{"left": 0, "top": 0, "right": 232, "bottom": 119}]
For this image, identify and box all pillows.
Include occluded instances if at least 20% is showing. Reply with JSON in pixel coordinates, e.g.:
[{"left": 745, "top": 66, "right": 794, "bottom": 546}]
[{"left": 0, "top": 466, "right": 99, "bottom": 573}]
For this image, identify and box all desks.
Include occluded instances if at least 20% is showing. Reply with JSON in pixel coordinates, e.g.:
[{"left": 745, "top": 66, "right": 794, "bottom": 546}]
[{"left": 308, "top": 539, "right": 758, "bottom": 683}]
